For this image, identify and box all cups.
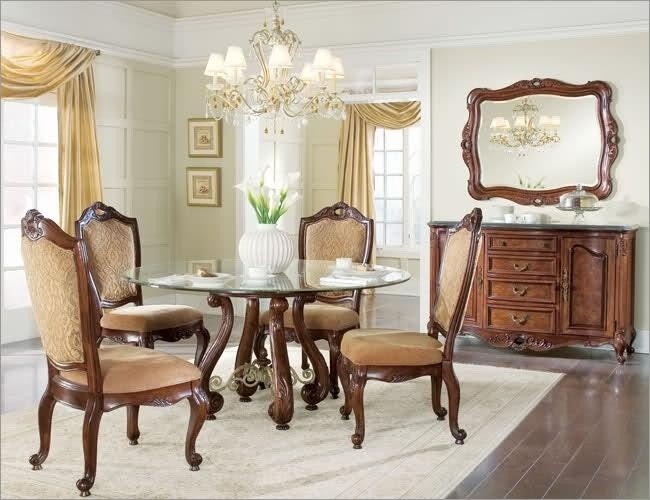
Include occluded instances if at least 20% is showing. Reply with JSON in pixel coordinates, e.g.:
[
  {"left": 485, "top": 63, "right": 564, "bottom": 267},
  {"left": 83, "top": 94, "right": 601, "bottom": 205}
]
[
  {"left": 502, "top": 214, "right": 539, "bottom": 225},
  {"left": 335, "top": 257, "right": 352, "bottom": 275}
]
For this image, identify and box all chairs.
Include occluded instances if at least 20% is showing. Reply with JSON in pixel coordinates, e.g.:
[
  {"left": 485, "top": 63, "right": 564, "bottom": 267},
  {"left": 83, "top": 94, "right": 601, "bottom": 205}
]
[
  {"left": 252, "top": 202, "right": 375, "bottom": 400},
  {"left": 74, "top": 204, "right": 209, "bottom": 368},
  {"left": 339, "top": 208, "right": 482, "bottom": 449},
  {"left": 21, "top": 208, "right": 211, "bottom": 498}
]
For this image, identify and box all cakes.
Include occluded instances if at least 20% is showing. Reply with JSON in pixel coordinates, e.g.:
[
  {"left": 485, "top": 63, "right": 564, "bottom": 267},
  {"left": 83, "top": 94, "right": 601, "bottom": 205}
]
[{"left": 565, "top": 198, "right": 590, "bottom": 208}]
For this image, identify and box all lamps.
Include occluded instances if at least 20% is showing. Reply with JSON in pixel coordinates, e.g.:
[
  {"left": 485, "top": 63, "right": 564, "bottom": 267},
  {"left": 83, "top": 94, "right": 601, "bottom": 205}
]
[
  {"left": 488, "top": 101, "right": 563, "bottom": 146},
  {"left": 199, "top": 1, "right": 344, "bottom": 125}
]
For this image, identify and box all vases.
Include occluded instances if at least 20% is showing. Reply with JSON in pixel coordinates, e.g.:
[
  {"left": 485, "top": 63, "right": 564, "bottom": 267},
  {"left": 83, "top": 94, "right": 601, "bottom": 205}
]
[{"left": 237, "top": 224, "right": 295, "bottom": 273}]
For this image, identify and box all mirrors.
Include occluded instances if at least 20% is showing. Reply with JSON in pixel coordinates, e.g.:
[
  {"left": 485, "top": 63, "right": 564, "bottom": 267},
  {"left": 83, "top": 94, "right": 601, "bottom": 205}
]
[{"left": 461, "top": 78, "right": 620, "bottom": 206}]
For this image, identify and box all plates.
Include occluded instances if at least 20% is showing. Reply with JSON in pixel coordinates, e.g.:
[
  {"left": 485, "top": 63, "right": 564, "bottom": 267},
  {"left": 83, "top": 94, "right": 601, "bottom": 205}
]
[{"left": 180, "top": 271, "right": 234, "bottom": 285}]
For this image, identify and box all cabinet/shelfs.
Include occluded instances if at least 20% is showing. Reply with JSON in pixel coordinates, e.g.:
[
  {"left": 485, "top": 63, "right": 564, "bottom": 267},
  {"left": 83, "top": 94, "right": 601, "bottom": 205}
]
[{"left": 427, "top": 220, "right": 639, "bottom": 365}]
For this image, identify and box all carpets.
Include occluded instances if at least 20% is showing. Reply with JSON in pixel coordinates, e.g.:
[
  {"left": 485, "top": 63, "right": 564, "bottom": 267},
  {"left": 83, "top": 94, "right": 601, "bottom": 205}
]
[{"left": 0, "top": 346, "right": 565, "bottom": 499}]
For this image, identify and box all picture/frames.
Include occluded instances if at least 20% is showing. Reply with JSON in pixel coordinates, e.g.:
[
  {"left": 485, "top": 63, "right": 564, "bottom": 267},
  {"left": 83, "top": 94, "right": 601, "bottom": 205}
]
[
  {"left": 187, "top": 167, "right": 221, "bottom": 206},
  {"left": 188, "top": 117, "right": 222, "bottom": 157}
]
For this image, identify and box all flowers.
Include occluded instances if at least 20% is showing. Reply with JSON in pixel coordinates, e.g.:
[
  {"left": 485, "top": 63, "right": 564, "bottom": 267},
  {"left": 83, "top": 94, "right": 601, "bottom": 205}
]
[{"left": 230, "top": 164, "right": 304, "bottom": 221}]
